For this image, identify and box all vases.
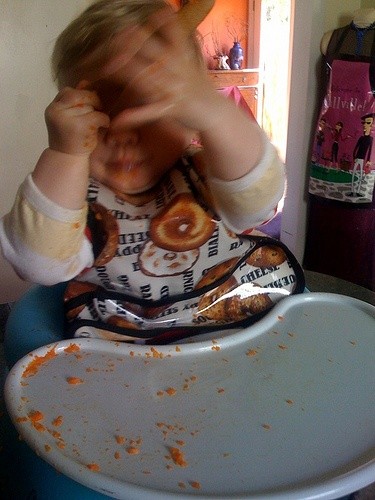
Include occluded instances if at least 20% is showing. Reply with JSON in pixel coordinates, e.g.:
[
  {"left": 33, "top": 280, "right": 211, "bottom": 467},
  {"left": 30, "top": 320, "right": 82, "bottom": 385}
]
[{"left": 229, "top": 42, "right": 243, "bottom": 70}]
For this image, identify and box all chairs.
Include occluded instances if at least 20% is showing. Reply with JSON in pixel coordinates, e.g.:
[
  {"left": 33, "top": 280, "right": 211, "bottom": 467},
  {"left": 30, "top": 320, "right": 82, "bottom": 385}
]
[{"left": 0, "top": 280, "right": 353, "bottom": 500}]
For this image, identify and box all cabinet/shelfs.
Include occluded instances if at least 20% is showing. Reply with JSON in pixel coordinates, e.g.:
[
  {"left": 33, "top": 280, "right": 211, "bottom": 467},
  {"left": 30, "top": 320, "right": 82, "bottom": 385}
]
[{"left": 209, "top": 70, "right": 259, "bottom": 121}]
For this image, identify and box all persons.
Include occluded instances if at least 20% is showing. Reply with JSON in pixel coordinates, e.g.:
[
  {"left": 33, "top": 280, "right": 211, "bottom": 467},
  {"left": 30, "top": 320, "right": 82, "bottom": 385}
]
[{"left": 0, "top": 1, "right": 312, "bottom": 344}]
